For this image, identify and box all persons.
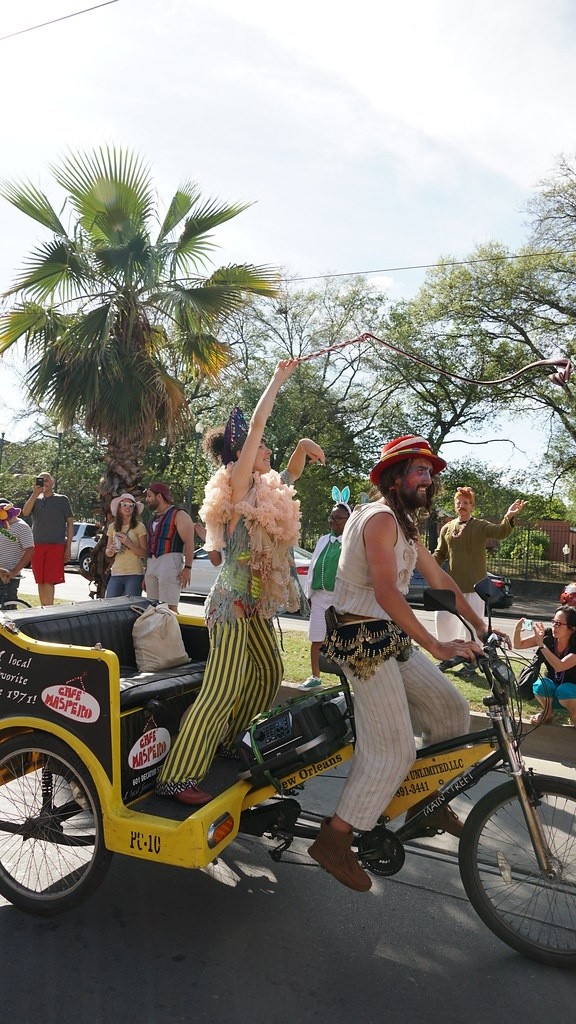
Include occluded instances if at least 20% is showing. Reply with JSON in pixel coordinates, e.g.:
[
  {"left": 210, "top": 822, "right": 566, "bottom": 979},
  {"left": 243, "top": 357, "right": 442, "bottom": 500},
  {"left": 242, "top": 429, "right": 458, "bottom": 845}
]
[
  {"left": 0, "top": 498, "right": 35, "bottom": 610},
  {"left": 105, "top": 483, "right": 194, "bottom": 612},
  {"left": 160, "top": 358, "right": 326, "bottom": 804},
  {"left": 304, "top": 434, "right": 465, "bottom": 891},
  {"left": 192, "top": 523, "right": 222, "bottom": 567},
  {"left": 515, "top": 606, "right": 576, "bottom": 728},
  {"left": 303, "top": 505, "right": 352, "bottom": 684},
  {"left": 434, "top": 487, "right": 529, "bottom": 676},
  {"left": 22, "top": 472, "right": 74, "bottom": 606}
]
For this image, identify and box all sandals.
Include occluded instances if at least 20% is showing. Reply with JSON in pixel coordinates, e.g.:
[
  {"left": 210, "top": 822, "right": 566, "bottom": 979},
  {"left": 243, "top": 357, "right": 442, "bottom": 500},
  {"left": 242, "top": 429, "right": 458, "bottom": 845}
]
[
  {"left": 530, "top": 712, "right": 554, "bottom": 724},
  {"left": 562, "top": 718, "right": 576, "bottom": 727}
]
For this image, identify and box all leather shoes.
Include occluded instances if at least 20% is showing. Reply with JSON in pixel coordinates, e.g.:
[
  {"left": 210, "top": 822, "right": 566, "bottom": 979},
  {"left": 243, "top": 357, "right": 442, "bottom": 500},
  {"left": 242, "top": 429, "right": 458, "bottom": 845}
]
[{"left": 174, "top": 785, "right": 214, "bottom": 805}]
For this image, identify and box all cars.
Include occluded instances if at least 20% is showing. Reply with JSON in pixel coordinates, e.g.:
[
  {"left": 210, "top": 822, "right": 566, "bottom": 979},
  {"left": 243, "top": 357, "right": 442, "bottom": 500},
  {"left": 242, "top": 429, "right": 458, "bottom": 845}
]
[
  {"left": 181, "top": 545, "right": 314, "bottom": 615},
  {"left": 404, "top": 559, "right": 514, "bottom": 616}
]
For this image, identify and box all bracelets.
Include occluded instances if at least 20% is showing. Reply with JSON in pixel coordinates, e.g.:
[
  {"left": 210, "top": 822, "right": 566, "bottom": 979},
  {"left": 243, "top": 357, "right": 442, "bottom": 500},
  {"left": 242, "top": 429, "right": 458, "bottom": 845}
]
[
  {"left": 185, "top": 565, "right": 192, "bottom": 569},
  {"left": 540, "top": 645, "right": 546, "bottom": 649}
]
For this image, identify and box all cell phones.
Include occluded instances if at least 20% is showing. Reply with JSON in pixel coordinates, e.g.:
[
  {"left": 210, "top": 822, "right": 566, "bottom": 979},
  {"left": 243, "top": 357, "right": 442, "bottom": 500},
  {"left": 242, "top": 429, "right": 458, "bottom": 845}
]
[
  {"left": 35, "top": 478, "right": 45, "bottom": 487},
  {"left": 522, "top": 620, "right": 533, "bottom": 631}
]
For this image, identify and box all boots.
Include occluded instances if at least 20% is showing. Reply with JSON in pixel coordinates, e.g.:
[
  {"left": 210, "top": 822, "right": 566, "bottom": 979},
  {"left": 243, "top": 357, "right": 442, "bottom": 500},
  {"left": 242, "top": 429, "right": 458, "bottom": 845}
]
[
  {"left": 307, "top": 817, "right": 372, "bottom": 892},
  {"left": 405, "top": 789, "right": 464, "bottom": 838}
]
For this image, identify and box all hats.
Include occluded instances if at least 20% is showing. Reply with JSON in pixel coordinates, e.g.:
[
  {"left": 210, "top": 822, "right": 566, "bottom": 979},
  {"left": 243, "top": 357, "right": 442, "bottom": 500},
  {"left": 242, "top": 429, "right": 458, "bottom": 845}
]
[
  {"left": 110, "top": 496, "right": 144, "bottom": 517},
  {"left": 370, "top": 436, "right": 446, "bottom": 487},
  {"left": 0, "top": 503, "right": 21, "bottom": 528},
  {"left": 149, "top": 484, "right": 172, "bottom": 503}
]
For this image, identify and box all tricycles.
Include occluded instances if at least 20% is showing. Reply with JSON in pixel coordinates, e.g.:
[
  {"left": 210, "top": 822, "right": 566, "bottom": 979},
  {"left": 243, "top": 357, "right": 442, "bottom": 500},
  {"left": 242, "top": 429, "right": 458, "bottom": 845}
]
[{"left": 0, "top": 576, "right": 576, "bottom": 971}]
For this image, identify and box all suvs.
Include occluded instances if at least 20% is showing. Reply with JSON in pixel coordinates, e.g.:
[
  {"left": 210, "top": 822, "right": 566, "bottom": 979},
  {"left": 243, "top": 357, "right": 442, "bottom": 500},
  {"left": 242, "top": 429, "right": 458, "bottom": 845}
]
[{"left": 23, "top": 522, "right": 105, "bottom": 574}]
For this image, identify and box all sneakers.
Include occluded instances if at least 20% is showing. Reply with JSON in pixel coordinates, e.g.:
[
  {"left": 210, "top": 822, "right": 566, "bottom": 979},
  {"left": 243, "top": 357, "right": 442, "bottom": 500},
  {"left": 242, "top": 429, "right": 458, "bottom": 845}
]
[{"left": 297, "top": 675, "right": 322, "bottom": 691}]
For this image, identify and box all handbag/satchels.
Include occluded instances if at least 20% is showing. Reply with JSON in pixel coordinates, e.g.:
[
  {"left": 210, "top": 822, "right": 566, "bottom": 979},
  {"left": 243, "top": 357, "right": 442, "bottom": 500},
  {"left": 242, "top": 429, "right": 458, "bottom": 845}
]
[{"left": 130, "top": 601, "right": 191, "bottom": 673}]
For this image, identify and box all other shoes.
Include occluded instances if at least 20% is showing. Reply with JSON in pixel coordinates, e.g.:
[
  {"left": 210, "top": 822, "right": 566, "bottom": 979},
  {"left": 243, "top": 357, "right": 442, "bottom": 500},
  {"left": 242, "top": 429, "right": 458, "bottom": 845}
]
[{"left": 454, "top": 667, "right": 478, "bottom": 678}]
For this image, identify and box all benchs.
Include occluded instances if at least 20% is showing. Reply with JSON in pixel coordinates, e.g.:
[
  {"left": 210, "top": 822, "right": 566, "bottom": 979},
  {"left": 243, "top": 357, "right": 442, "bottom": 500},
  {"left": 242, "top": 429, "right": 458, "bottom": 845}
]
[{"left": 0, "top": 594, "right": 211, "bottom": 712}]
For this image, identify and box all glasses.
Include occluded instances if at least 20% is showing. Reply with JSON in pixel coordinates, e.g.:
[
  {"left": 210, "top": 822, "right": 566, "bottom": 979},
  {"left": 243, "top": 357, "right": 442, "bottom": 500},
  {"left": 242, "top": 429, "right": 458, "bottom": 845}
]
[
  {"left": 328, "top": 515, "right": 348, "bottom": 522},
  {"left": 120, "top": 502, "right": 136, "bottom": 508},
  {"left": 552, "top": 619, "right": 567, "bottom": 627}
]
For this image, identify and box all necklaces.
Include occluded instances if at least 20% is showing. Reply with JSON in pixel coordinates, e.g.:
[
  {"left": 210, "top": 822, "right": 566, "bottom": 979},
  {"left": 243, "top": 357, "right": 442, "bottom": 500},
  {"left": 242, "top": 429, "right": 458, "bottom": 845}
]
[{"left": 451, "top": 514, "right": 473, "bottom": 537}]
